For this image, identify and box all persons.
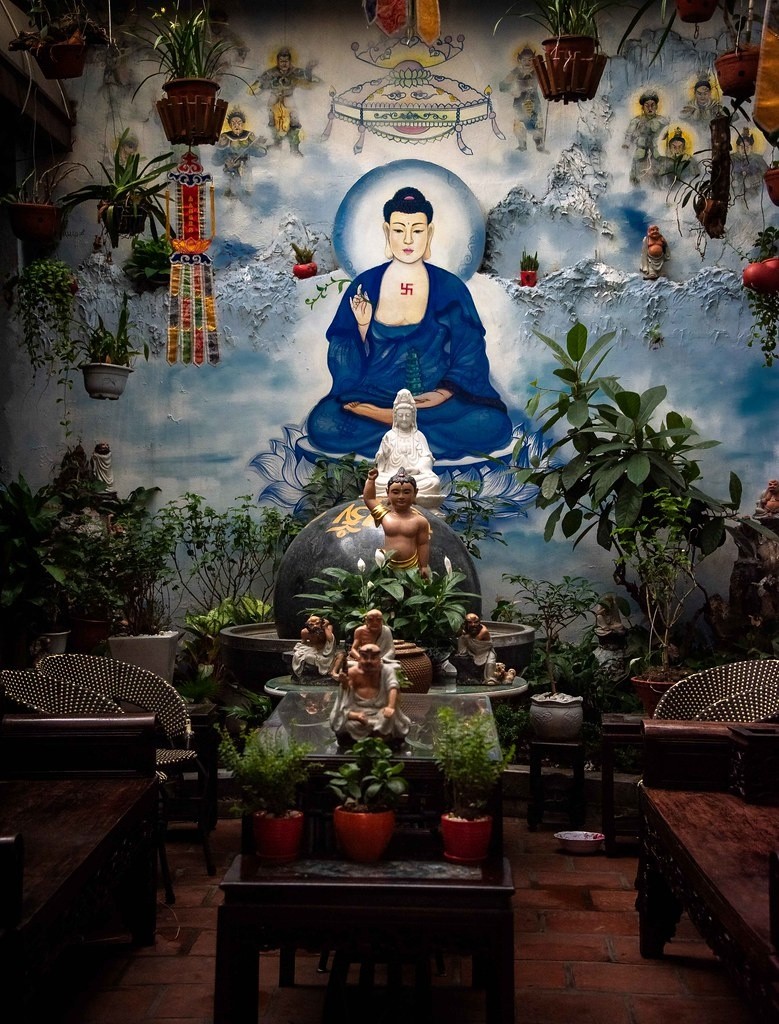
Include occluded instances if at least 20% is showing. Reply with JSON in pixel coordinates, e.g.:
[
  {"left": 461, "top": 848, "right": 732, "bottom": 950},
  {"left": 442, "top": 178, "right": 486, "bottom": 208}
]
[
  {"left": 597, "top": 595, "right": 624, "bottom": 631},
  {"left": 363, "top": 467, "right": 430, "bottom": 582},
  {"left": 755, "top": 480, "right": 779, "bottom": 516},
  {"left": 292, "top": 616, "right": 347, "bottom": 681},
  {"left": 374, "top": 389, "right": 441, "bottom": 495},
  {"left": 329, "top": 644, "right": 412, "bottom": 741},
  {"left": 346, "top": 609, "right": 401, "bottom": 670},
  {"left": 639, "top": 225, "right": 670, "bottom": 278},
  {"left": 455, "top": 613, "right": 500, "bottom": 685},
  {"left": 90, "top": 443, "right": 114, "bottom": 488}
]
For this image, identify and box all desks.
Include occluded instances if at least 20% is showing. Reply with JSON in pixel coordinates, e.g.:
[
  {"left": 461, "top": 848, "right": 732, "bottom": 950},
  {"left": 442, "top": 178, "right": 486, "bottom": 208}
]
[
  {"left": 599, "top": 713, "right": 652, "bottom": 853},
  {"left": 120, "top": 698, "right": 217, "bottom": 834},
  {"left": 527, "top": 735, "right": 585, "bottom": 831},
  {"left": 212, "top": 848, "right": 516, "bottom": 1024}
]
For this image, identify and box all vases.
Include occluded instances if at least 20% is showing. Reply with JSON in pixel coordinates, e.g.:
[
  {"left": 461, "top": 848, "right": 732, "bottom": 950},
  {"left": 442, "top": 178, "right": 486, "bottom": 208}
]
[
  {"left": 160, "top": 77, "right": 221, "bottom": 112},
  {"left": 542, "top": 37, "right": 595, "bottom": 83},
  {"left": 713, "top": 50, "right": 758, "bottom": 93}
]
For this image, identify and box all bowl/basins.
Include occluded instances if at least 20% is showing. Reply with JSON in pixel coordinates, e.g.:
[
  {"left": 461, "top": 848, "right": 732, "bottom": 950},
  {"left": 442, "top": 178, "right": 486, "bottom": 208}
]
[{"left": 553, "top": 831, "right": 605, "bottom": 852}]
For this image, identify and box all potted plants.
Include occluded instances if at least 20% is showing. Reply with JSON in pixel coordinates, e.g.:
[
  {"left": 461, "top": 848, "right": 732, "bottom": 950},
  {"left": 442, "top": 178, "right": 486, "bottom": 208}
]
[
  {"left": 28, "top": 0, "right": 92, "bottom": 80},
  {"left": 1, "top": 162, "right": 94, "bottom": 243},
  {"left": 106, "top": 510, "right": 214, "bottom": 689},
  {"left": 209, "top": 711, "right": 329, "bottom": 867},
  {"left": 503, "top": 573, "right": 606, "bottom": 743},
  {"left": 520, "top": 250, "right": 540, "bottom": 288},
  {"left": 60, "top": 127, "right": 180, "bottom": 250},
  {"left": 429, "top": 704, "right": 516, "bottom": 865},
  {"left": 64, "top": 581, "right": 119, "bottom": 654},
  {"left": 760, "top": 160, "right": 779, "bottom": 207},
  {"left": 322, "top": 736, "right": 409, "bottom": 862},
  {"left": 65, "top": 287, "right": 151, "bottom": 400},
  {"left": 727, "top": 225, "right": 779, "bottom": 368},
  {"left": 36, "top": 562, "right": 72, "bottom": 654},
  {"left": 617, "top": 0, "right": 720, "bottom": 68},
  {"left": 609, "top": 486, "right": 696, "bottom": 719},
  {"left": 291, "top": 242, "right": 320, "bottom": 283}
]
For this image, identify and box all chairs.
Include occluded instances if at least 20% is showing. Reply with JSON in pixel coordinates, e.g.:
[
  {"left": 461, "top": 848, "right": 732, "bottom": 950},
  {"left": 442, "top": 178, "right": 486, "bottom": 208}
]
[
  {"left": 0, "top": 653, "right": 217, "bottom": 902},
  {"left": 651, "top": 658, "right": 779, "bottom": 723}
]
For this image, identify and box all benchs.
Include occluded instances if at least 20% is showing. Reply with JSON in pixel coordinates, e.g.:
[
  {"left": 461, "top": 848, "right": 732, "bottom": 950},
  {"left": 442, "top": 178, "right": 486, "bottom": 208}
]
[
  {"left": 0, "top": 712, "right": 162, "bottom": 1024},
  {"left": 634, "top": 720, "right": 779, "bottom": 1024}
]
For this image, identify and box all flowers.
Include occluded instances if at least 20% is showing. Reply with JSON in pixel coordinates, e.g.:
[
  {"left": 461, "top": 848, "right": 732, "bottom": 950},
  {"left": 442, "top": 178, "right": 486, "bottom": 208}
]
[
  {"left": 490, "top": 1, "right": 644, "bottom": 57},
  {"left": 120, "top": 0, "right": 256, "bottom": 101}
]
